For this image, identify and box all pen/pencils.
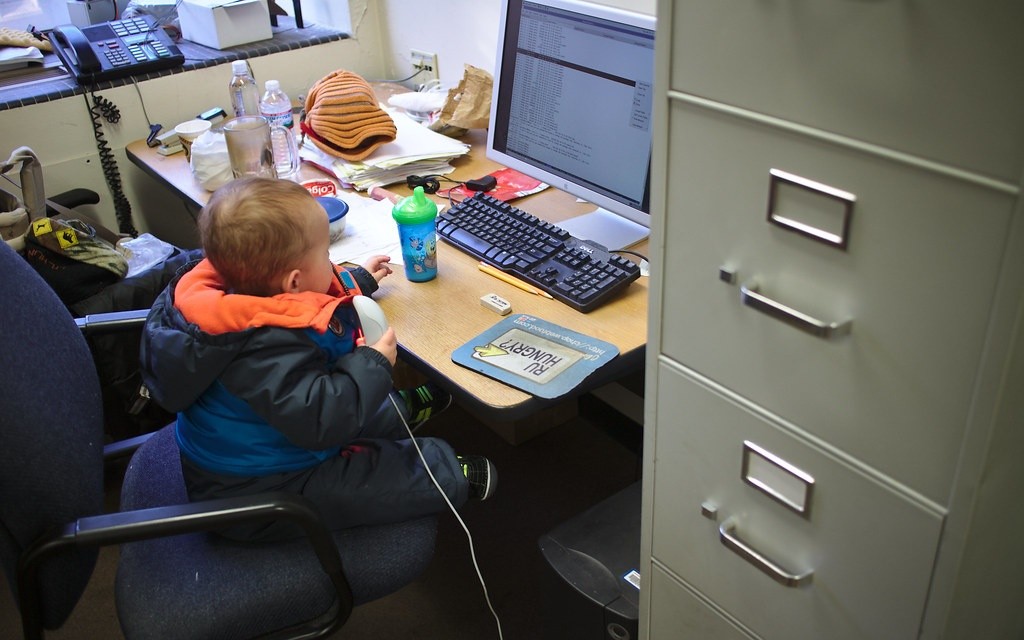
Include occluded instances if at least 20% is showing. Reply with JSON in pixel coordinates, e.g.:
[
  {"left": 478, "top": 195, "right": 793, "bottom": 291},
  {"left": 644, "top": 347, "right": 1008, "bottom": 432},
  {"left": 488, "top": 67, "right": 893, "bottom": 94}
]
[
  {"left": 478, "top": 264, "right": 539, "bottom": 295},
  {"left": 479, "top": 260, "right": 554, "bottom": 299}
]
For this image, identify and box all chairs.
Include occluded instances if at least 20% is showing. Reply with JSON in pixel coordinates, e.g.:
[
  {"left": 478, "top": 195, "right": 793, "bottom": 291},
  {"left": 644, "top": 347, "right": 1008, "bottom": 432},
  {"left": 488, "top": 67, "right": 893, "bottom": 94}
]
[{"left": 0, "top": 243, "right": 463, "bottom": 640}]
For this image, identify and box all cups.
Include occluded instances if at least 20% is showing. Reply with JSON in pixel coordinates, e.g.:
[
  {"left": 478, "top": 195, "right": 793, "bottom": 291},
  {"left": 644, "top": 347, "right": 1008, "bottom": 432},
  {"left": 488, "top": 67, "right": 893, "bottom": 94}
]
[
  {"left": 221, "top": 115, "right": 299, "bottom": 182},
  {"left": 174, "top": 120, "right": 213, "bottom": 163}
]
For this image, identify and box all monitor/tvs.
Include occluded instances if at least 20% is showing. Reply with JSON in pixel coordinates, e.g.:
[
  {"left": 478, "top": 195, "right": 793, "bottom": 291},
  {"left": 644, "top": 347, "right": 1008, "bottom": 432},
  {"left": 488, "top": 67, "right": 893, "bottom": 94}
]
[{"left": 486, "top": 0, "right": 657, "bottom": 229}]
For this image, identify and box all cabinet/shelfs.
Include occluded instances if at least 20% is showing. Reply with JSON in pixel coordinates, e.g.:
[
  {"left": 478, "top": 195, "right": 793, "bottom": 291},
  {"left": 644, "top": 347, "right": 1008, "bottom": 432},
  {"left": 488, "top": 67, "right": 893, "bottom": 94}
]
[{"left": 639, "top": 0, "right": 1024, "bottom": 640}]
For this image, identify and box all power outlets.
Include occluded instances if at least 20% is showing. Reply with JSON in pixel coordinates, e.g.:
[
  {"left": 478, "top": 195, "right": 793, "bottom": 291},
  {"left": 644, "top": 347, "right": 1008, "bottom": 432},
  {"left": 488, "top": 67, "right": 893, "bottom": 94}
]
[{"left": 408, "top": 46, "right": 439, "bottom": 91}]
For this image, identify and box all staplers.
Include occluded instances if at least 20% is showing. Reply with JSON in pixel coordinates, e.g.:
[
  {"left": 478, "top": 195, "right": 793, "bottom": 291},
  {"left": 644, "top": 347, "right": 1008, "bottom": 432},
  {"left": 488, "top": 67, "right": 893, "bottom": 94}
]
[{"left": 156, "top": 108, "right": 226, "bottom": 154}]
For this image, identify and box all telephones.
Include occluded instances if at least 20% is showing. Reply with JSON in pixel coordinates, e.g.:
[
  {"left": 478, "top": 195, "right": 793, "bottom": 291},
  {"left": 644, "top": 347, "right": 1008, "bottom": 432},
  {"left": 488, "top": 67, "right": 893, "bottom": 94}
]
[{"left": 47, "top": 15, "right": 185, "bottom": 87}]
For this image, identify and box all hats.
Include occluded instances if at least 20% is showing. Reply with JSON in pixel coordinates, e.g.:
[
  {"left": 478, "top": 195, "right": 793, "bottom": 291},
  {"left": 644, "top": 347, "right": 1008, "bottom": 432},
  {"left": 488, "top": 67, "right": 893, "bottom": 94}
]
[
  {"left": 299, "top": 71, "right": 396, "bottom": 161},
  {"left": 24, "top": 217, "right": 128, "bottom": 305}
]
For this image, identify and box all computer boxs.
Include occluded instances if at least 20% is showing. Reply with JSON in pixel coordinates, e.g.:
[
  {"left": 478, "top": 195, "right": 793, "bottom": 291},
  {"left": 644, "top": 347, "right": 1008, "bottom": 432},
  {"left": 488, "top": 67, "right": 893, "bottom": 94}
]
[{"left": 535, "top": 478, "right": 643, "bottom": 640}]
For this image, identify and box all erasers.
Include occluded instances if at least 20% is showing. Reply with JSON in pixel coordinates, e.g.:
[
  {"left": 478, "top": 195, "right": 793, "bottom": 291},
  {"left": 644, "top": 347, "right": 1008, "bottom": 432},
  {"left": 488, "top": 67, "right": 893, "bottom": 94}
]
[{"left": 480, "top": 293, "right": 512, "bottom": 317}]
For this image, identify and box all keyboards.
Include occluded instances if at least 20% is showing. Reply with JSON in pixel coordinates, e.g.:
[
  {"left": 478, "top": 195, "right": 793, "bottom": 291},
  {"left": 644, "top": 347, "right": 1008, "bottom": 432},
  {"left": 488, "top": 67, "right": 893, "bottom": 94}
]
[{"left": 435, "top": 191, "right": 641, "bottom": 313}]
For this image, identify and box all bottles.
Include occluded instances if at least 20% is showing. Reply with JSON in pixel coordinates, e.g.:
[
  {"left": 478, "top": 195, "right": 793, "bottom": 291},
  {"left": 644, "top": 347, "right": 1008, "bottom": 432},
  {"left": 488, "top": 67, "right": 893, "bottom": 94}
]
[
  {"left": 258, "top": 80, "right": 297, "bottom": 176},
  {"left": 392, "top": 186, "right": 439, "bottom": 282},
  {"left": 227, "top": 59, "right": 259, "bottom": 119}
]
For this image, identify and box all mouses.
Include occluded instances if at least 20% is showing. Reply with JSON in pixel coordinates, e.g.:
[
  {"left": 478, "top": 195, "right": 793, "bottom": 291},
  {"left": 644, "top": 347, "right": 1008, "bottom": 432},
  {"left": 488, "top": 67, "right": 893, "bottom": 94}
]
[{"left": 353, "top": 295, "right": 388, "bottom": 346}]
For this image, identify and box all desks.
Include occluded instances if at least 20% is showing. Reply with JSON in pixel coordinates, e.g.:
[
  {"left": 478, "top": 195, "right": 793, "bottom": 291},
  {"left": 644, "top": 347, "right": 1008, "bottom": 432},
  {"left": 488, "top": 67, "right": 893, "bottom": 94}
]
[{"left": 128, "top": 79, "right": 651, "bottom": 416}]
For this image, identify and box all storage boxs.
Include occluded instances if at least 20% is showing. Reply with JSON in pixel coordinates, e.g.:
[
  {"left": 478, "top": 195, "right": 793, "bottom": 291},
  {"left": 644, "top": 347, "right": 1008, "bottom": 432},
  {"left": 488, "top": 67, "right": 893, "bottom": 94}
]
[{"left": 175, "top": 0, "right": 273, "bottom": 50}]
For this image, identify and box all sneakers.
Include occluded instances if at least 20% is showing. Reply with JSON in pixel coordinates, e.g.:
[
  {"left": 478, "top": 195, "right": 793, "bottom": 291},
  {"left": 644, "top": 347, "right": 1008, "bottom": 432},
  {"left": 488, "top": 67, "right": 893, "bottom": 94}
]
[
  {"left": 456, "top": 455, "right": 498, "bottom": 500},
  {"left": 399, "top": 382, "right": 452, "bottom": 433}
]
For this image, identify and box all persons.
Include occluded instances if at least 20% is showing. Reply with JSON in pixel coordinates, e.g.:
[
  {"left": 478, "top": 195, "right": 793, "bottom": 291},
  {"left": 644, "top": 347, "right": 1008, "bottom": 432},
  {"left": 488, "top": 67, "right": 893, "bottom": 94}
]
[{"left": 141, "top": 177, "right": 497, "bottom": 544}]
